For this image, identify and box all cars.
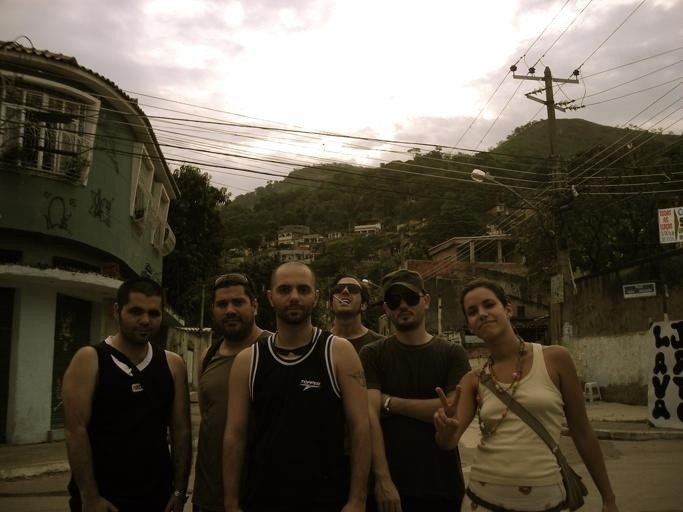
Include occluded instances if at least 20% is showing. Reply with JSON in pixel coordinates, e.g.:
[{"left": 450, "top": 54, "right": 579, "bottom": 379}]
[{"left": 515, "top": 314, "right": 549, "bottom": 342}]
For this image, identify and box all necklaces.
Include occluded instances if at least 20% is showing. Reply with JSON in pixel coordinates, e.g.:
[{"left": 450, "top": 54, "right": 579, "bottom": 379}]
[{"left": 474, "top": 335, "right": 527, "bottom": 437}]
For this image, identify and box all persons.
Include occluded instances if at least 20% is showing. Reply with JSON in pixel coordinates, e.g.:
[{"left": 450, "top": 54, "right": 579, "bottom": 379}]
[
  {"left": 222, "top": 262, "right": 371, "bottom": 510},
  {"left": 190, "top": 274, "right": 274, "bottom": 511},
  {"left": 328, "top": 272, "right": 386, "bottom": 353},
  {"left": 433, "top": 279, "right": 620, "bottom": 511},
  {"left": 63, "top": 276, "right": 191, "bottom": 510},
  {"left": 359, "top": 268, "right": 472, "bottom": 512}
]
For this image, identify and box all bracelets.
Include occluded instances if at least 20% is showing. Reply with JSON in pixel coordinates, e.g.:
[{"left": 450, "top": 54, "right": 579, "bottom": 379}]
[
  {"left": 172, "top": 489, "right": 189, "bottom": 504},
  {"left": 224, "top": 498, "right": 238, "bottom": 502}
]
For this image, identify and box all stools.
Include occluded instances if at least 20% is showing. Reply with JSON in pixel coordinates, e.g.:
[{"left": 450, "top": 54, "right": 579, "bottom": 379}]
[{"left": 584, "top": 382, "right": 602, "bottom": 404}]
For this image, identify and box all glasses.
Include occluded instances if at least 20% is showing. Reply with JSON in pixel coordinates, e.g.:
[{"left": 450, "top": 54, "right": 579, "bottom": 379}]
[
  {"left": 272, "top": 327, "right": 313, "bottom": 355},
  {"left": 387, "top": 293, "right": 424, "bottom": 310},
  {"left": 334, "top": 284, "right": 362, "bottom": 293}
]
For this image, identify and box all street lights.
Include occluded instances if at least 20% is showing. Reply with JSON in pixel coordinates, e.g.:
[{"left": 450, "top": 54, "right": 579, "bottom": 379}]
[
  {"left": 470, "top": 169, "right": 549, "bottom": 219},
  {"left": 361, "top": 278, "right": 382, "bottom": 289}
]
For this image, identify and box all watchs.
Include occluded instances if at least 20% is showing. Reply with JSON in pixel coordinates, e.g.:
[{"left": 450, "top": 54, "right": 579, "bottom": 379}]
[{"left": 380, "top": 396, "right": 392, "bottom": 416}]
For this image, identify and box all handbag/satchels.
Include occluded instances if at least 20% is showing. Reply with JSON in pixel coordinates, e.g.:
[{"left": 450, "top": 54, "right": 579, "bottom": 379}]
[{"left": 555, "top": 448, "right": 588, "bottom": 512}]
